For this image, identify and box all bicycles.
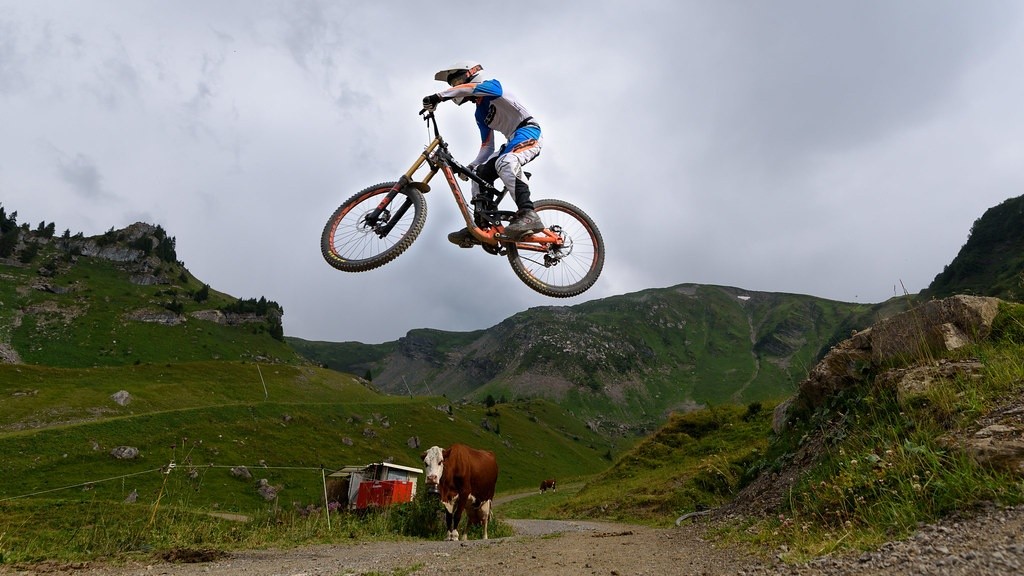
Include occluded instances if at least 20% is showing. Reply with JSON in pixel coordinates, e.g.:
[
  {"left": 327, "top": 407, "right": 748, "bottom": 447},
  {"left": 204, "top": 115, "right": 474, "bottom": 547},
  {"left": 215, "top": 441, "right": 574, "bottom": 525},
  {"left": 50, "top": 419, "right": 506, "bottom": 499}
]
[{"left": 320, "top": 101, "right": 606, "bottom": 299}]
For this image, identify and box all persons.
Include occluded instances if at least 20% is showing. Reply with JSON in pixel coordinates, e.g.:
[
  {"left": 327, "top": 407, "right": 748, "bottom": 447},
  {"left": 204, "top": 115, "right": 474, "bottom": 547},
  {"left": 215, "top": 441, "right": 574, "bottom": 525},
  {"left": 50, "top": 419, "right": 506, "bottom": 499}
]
[{"left": 422, "top": 59, "right": 545, "bottom": 248}]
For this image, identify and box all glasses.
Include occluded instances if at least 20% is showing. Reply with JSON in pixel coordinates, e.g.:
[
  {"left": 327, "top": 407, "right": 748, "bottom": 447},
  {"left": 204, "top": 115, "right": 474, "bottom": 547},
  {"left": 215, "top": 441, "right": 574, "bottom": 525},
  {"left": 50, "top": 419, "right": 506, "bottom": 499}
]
[{"left": 451, "top": 77, "right": 463, "bottom": 89}]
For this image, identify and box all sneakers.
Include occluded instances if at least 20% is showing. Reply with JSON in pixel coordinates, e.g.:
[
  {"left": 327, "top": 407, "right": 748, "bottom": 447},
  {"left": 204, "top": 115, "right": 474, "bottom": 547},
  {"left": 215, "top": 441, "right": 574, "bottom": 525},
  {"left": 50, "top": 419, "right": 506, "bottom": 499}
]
[
  {"left": 504, "top": 208, "right": 545, "bottom": 239},
  {"left": 447, "top": 226, "right": 482, "bottom": 245}
]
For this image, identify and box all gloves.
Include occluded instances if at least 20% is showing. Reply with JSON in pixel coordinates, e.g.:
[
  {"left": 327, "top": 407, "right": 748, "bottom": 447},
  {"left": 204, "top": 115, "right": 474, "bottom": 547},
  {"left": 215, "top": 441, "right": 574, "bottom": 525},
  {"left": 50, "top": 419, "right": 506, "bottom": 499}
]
[
  {"left": 458, "top": 166, "right": 471, "bottom": 182},
  {"left": 423, "top": 94, "right": 441, "bottom": 112}
]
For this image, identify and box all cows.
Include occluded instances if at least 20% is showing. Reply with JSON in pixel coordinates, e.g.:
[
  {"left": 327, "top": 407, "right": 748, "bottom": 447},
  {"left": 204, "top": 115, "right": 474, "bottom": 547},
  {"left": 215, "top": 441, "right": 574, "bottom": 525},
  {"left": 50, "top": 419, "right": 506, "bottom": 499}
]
[
  {"left": 420, "top": 443, "right": 497, "bottom": 540},
  {"left": 539, "top": 479, "right": 556, "bottom": 494}
]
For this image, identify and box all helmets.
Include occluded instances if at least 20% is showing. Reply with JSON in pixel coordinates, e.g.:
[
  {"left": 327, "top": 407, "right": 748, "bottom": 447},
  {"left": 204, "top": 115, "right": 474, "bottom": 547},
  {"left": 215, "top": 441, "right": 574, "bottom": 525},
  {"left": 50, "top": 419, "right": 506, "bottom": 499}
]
[{"left": 434, "top": 59, "right": 488, "bottom": 106}]
